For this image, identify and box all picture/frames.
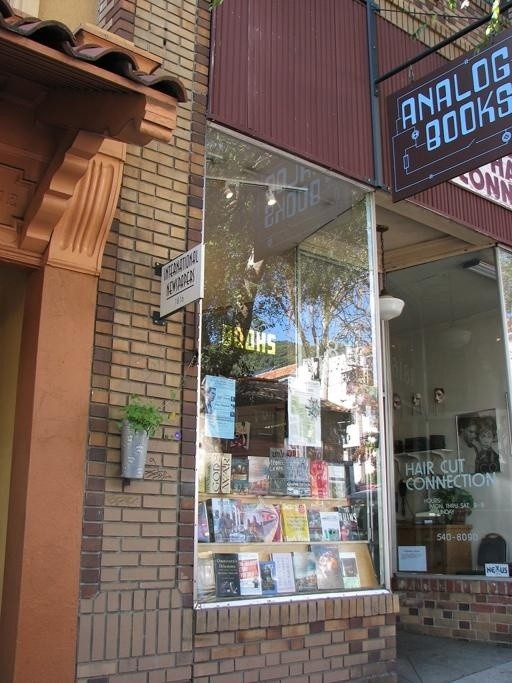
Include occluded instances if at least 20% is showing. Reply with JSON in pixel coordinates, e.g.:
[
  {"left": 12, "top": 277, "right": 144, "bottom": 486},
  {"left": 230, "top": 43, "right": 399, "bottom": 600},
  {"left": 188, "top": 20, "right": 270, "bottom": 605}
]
[{"left": 456, "top": 408, "right": 501, "bottom": 476}]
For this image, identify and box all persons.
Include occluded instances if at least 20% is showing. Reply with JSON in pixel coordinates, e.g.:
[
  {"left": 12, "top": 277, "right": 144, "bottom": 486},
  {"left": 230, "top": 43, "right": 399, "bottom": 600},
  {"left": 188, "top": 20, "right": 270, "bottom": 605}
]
[
  {"left": 203, "top": 386, "right": 216, "bottom": 415},
  {"left": 457, "top": 412, "right": 481, "bottom": 474},
  {"left": 474, "top": 414, "right": 499, "bottom": 473}
]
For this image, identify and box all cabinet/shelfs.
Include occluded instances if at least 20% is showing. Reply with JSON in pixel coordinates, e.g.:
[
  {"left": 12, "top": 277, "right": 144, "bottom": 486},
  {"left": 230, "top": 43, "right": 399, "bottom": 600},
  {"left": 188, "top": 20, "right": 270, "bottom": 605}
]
[{"left": 197, "top": 491, "right": 381, "bottom": 603}]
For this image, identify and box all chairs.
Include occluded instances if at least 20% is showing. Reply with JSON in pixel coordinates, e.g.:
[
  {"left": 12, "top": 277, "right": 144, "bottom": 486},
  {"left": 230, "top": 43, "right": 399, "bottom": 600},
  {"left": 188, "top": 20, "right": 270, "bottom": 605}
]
[{"left": 457, "top": 533, "right": 506, "bottom": 574}]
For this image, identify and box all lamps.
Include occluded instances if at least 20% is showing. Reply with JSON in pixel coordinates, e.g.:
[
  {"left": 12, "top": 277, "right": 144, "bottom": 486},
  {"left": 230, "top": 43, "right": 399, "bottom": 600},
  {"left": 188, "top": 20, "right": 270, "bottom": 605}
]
[
  {"left": 375, "top": 224, "right": 405, "bottom": 322},
  {"left": 223, "top": 177, "right": 277, "bottom": 205},
  {"left": 464, "top": 258, "right": 497, "bottom": 281}
]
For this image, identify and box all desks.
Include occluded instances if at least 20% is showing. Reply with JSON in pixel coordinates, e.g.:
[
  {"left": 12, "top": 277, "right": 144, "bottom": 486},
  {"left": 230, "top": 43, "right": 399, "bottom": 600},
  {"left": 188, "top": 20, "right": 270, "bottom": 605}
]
[{"left": 396, "top": 523, "right": 473, "bottom": 574}]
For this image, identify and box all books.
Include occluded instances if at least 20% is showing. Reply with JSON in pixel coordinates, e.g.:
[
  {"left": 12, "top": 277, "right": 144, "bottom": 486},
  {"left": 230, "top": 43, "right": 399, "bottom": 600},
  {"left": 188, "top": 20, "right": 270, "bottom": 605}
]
[
  {"left": 292, "top": 550, "right": 318, "bottom": 593},
  {"left": 308, "top": 543, "right": 345, "bottom": 590},
  {"left": 238, "top": 551, "right": 264, "bottom": 596},
  {"left": 259, "top": 560, "right": 277, "bottom": 596},
  {"left": 197, "top": 447, "right": 360, "bottom": 544},
  {"left": 197, "top": 550, "right": 216, "bottom": 603},
  {"left": 269, "top": 552, "right": 296, "bottom": 595},
  {"left": 337, "top": 551, "right": 362, "bottom": 588},
  {"left": 213, "top": 552, "right": 241, "bottom": 598}
]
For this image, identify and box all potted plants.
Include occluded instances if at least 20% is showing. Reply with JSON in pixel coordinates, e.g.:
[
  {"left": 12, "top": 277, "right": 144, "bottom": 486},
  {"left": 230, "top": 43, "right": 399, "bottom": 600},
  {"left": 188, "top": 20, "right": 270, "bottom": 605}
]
[
  {"left": 117, "top": 388, "right": 177, "bottom": 481},
  {"left": 432, "top": 486, "right": 474, "bottom": 525}
]
